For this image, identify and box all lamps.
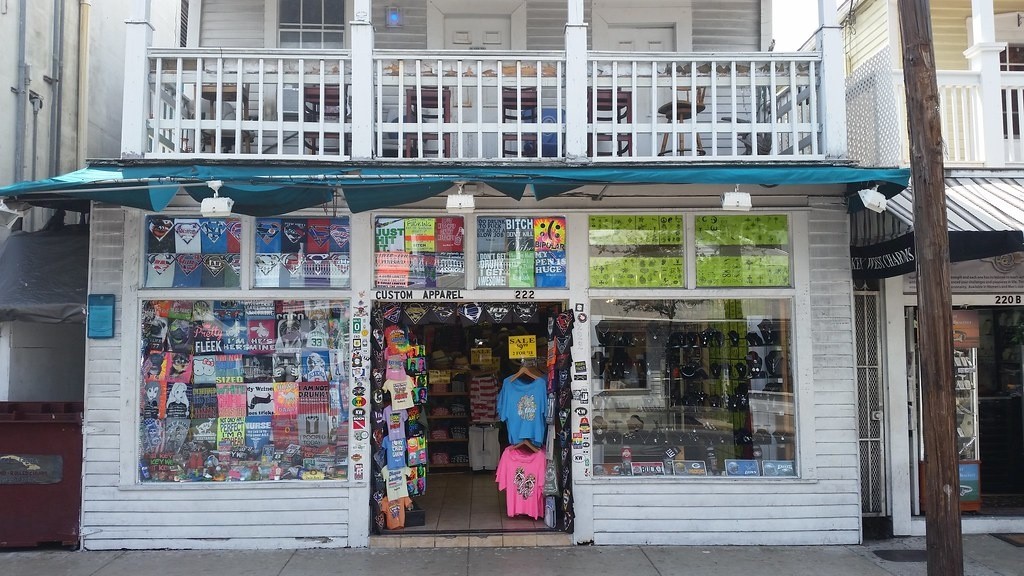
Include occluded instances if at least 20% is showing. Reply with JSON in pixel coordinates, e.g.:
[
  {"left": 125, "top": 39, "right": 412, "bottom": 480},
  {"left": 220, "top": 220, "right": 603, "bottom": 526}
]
[
  {"left": 858, "top": 182, "right": 889, "bottom": 213},
  {"left": 444, "top": 181, "right": 475, "bottom": 214},
  {"left": 199, "top": 179, "right": 234, "bottom": 219},
  {"left": 718, "top": 184, "right": 753, "bottom": 213}
]
